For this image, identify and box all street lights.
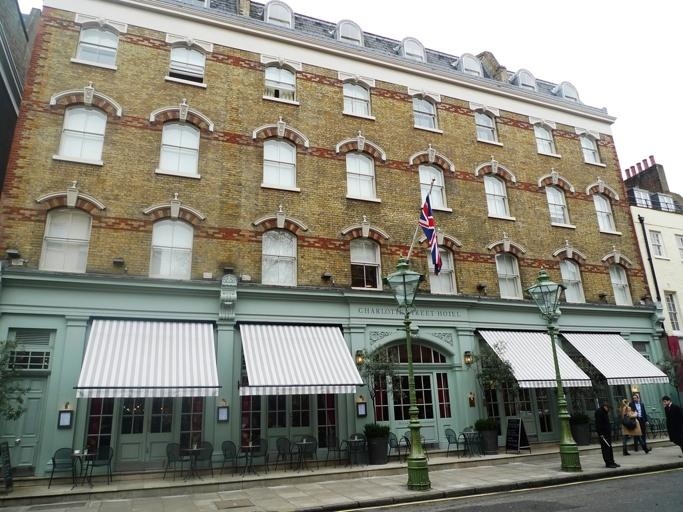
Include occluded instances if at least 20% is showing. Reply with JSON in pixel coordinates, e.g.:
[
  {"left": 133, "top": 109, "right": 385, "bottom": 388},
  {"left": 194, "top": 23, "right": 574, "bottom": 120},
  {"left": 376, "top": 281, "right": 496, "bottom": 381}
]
[
  {"left": 524, "top": 270, "right": 583, "bottom": 473},
  {"left": 386, "top": 255, "right": 432, "bottom": 490}
]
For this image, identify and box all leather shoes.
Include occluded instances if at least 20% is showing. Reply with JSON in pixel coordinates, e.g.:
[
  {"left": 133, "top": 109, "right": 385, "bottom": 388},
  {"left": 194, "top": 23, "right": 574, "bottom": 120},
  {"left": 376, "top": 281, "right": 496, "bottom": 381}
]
[
  {"left": 623, "top": 450, "right": 630, "bottom": 456},
  {"left": 643, "top": 446, "right": 652, "bottom": 452},
  {"left": 605, "top": 463, "right": 617, "bottom": 468},
  {"left": 614, "top": 463, "right": 620, "bottom": 467}
]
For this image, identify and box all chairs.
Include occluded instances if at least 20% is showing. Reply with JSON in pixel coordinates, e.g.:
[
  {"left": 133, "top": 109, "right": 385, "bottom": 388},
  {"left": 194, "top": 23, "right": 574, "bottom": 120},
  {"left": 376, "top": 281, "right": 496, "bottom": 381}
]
[
  {"left": 350, "top": 432, "right": 366, "bottom": 464},
  {"left": 47, "top": 448, "right": 75, "bottom": 488},
  {"left": 83, "top": 446, "right": 113, "bottom": 485},
  {"left": 388, "top": 427, "right": 481, "bottom": 464},
  {"left": 193, "top": 441, "right": 214, "bottom": 479},
  {"left": 588, "top": 415, "right": 666, "bottom": 439},
  {"left": 274, "top": 437, "right": 296, "bottom": 472},
  {"left": 251, "top": 439, "right": 269, "bottom": 474},
  {"left": 162, "top": 443, "right": 188, "bottom": 481},
  {"left": 323, "top": 435, "right": 349, "bottom": 467},
  {"left": 218, "top": 440, "right": 237, "bottom": 476},
  {"left": 300, "top": 436, "right": 319, "bottom": 470}
]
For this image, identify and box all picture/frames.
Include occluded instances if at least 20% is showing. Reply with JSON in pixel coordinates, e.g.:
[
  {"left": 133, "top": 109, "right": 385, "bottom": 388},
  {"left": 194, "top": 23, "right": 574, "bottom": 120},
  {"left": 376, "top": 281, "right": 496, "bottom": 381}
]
[
  {"left": 355, "top": 402, "right": 366, "bottom": 418},
  {"left": 216, "top": 406, "right": 228, "bottom": 424},
  {"left": 56, "top": 410, "right": 73, "bottom": 429}
]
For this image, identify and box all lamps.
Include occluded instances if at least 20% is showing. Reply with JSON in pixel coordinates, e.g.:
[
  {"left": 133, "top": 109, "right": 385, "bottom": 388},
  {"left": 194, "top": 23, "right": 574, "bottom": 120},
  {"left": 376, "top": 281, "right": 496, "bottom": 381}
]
[
  {"left": 464, "top": 350, "right": 473, "bottom": 365},
  {"left": 354, "top": 350, "right": 364, "bottom": 367}
]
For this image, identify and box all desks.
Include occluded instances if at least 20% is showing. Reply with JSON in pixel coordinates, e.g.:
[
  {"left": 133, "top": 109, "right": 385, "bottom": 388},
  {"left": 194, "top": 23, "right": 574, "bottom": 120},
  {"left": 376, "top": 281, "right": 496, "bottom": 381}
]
[
  {"left": 341, "top": 440, "right": 359, "bottom": 468},
  {"left": 71, "top": 453, "right": 94, "bottom": 490},
  {"left": 236, "top": 445, "right": 257, "bottom": 476},
  {"left": 177, "top": 449, "right": 205, "bottom": 481}
]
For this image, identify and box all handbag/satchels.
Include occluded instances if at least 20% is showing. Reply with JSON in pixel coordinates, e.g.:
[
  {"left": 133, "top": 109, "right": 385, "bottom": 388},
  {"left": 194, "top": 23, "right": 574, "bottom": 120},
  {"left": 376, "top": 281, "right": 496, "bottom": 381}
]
[{"left": 622, "top": 415, "right": 637, "bottom": 430}]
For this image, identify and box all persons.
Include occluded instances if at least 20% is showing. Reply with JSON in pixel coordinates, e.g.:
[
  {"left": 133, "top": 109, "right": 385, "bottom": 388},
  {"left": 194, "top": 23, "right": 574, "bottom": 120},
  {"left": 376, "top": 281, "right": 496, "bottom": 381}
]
[
  {"left": 661, "top": 395, "right": 682, "bottom": 458},
  {"left": 617, "top": 392, "right": 652, "bottom": 456},
  {"left": 594, "top": 400, "right": 620, "bottom": 468}
]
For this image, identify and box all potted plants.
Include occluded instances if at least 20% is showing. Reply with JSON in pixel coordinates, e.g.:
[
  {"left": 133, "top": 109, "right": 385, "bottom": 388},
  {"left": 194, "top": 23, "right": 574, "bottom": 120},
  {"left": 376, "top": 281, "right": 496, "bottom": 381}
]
[
  {"left": 472, "top": 342, "right": 519, "bottom": 454},
  {"left": 359, "top": 346, "right": 404, "bottom": 465},
  {"left": 0, "top": 341, "right": 31, "bottom": 493}
]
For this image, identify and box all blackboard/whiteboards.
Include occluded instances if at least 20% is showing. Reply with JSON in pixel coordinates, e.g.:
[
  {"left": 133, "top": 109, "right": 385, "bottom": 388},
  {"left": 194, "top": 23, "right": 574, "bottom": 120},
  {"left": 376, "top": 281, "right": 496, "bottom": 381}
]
[
  {"left": 521, "top": 418, "right": 531, "bottom": 449},
  {"left": 506, "top": 418, "right": 521, "bottom": 451},
  {"left": 0, "top": 441, "right": 12, "bottom": 478}
]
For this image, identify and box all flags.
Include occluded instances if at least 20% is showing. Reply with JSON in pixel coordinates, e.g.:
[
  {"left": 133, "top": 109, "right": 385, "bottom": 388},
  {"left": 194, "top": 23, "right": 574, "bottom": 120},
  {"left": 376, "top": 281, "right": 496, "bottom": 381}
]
[{"left": 418, "top": 194, "right": 442, "bottom": 276}]
